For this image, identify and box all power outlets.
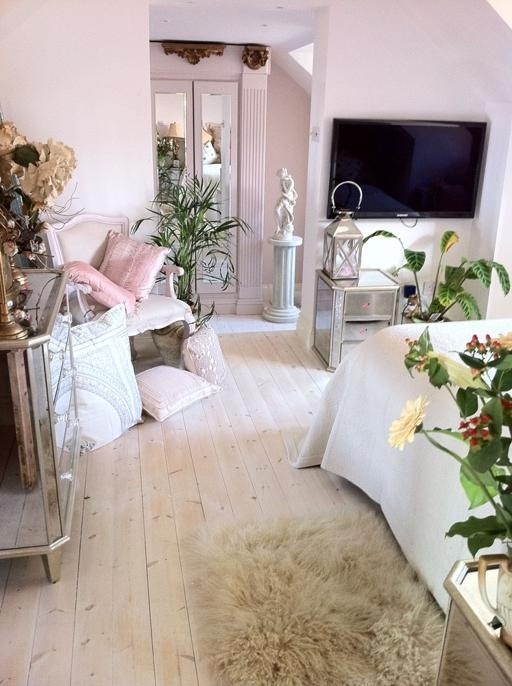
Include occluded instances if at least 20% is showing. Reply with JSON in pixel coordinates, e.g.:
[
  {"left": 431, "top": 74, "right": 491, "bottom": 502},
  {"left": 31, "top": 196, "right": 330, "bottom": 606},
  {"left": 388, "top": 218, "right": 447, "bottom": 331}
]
[{"left": 402, "top": 282, "right": 417, "bottom": 302}]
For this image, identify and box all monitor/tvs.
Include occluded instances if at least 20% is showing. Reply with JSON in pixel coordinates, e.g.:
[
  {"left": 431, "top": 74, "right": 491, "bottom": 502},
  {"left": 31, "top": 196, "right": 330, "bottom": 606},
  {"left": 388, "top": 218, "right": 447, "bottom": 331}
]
[{"left": 326, "top": 117, "right": 488, "bottom": 219}]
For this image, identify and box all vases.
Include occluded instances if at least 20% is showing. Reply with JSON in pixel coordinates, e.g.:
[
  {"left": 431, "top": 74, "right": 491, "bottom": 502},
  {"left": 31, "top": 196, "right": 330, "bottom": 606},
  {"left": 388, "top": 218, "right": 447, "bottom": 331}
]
[{"left": 479, "top": 551, "right": 512, "bottom": 648}]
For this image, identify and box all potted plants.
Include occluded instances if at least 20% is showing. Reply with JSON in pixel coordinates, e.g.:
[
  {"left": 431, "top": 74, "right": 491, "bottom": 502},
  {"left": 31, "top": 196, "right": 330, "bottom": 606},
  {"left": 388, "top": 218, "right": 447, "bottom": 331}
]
[
  {"left": 130, "top": 163, "right": 255, "bottom": 369},
  {"left": 363, "top": 230, "right": 510, "bottom": 323}
]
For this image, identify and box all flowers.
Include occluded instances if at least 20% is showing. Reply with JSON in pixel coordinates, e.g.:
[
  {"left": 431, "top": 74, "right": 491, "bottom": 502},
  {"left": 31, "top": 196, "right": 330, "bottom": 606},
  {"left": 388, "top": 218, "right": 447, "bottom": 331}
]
[
  {"left": 356, "top": 318, "right": 512, "bottom": 558},
  {"left": 0, "top": 120, "right": 85, "bottom": 273}
]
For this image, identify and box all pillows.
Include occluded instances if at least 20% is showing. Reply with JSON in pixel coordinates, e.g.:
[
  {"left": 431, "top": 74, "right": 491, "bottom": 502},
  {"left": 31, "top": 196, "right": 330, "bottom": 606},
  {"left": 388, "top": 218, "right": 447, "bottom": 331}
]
[{"left": 64, "top": 230, "right": 227, "bottom": 455}]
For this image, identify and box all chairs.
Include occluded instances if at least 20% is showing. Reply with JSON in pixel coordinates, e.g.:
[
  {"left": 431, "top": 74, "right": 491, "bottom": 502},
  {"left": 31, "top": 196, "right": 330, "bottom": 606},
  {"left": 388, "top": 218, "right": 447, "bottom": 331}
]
[{"left": 41, "top": 209, "right": 196, "bottom": 370}]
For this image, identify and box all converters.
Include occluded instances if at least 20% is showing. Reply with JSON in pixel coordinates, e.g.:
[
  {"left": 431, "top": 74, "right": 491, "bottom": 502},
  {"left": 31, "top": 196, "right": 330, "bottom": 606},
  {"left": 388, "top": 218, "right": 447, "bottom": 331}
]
[{"left": 404, "top": 285, "right": 416, "bottom": 298}]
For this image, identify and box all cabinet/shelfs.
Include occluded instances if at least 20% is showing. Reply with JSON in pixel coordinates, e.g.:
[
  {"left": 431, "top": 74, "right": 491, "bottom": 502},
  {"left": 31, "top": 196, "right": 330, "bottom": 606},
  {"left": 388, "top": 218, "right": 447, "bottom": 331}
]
[
  {"left": 434, "top": 558, "right": 512, "bottom": 686},
  {"left": 0, "top": 282, "right": 80, "bottom": 583},
  {"left": 315, "top": 268, "right": 401, "bottom": 372}
]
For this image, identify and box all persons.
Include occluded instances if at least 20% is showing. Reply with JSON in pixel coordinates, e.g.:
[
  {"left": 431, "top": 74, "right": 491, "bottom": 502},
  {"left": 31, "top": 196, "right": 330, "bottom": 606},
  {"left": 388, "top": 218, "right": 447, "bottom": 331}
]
[{"left": 274, "top": 167, "right": 297, "bottom": 235}]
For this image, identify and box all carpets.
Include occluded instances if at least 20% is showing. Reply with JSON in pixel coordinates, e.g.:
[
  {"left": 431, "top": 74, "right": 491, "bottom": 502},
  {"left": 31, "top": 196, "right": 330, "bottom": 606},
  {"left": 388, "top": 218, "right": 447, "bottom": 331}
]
[{"left": 184, "top": 510, "right": 446, "bottom": 686}]
